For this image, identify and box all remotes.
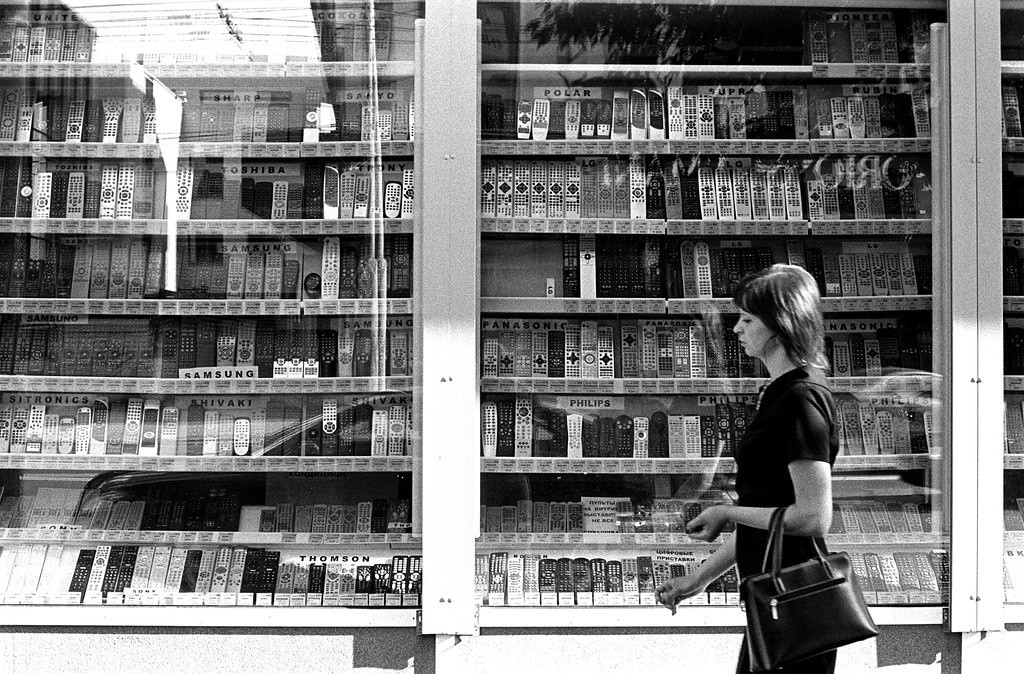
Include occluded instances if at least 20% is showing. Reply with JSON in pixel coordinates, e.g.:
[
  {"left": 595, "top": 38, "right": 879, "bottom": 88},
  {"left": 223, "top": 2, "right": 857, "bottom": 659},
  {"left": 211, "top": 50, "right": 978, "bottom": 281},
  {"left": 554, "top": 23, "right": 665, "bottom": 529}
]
[
  {"left": 0, "top": 18, "right": 423, "bottom": 604},
  {"left": 1001, "top": 85, "right": 1024, "bottom": 607},
  {"left": 476, "top": 15, "right": 955, "bottom": 611}
]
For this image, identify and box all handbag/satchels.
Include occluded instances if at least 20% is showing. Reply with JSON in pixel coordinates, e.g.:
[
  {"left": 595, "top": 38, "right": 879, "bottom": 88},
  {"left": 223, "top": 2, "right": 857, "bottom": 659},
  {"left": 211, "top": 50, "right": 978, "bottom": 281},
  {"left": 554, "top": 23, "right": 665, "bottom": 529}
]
[{"left": 739, "top": 507, "right": 880, "bottom": 674}]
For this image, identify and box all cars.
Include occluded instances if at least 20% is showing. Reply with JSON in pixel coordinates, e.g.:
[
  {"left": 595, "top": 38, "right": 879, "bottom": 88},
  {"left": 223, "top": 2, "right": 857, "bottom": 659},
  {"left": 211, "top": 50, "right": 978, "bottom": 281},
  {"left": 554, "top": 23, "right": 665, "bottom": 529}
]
[
  {"left": 68, "top": 383, "right": 737, "bottom": 549},
  {"left": 662, "top": 363, "right": 946, "bottom": 506}
]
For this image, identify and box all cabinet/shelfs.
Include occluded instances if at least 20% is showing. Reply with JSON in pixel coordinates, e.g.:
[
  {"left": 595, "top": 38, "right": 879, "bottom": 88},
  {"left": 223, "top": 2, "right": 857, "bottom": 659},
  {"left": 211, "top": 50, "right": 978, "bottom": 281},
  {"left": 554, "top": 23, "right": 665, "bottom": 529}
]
[
  {"left": 1001, "top": 60, "right": 1024, "bottom": 621},
  {"left": 0, "top": 62, "right": 424, "bottom": 631},
  {"left": 474, "top": 62, "right": 949, "bottom": 608}
]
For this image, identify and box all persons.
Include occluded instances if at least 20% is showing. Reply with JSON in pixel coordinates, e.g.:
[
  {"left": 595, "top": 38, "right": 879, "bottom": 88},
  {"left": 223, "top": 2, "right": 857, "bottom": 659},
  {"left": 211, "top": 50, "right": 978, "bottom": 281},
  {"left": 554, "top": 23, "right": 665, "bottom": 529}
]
[
  {"left": 655, "top": 262, "right": 838, "bottom": 674},
  {"left": 655, "top": 300, "right": 741, "bottom": 516}
]
